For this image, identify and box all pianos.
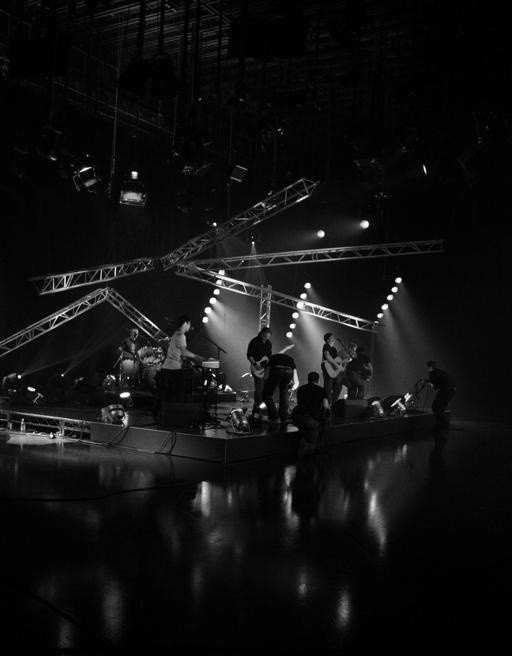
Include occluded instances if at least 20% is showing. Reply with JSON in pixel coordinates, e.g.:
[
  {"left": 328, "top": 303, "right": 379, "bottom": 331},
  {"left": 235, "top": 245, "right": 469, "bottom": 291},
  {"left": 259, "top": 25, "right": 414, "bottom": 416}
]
[{"left": 196, "top": 356, "right": 220, "bottom": 368}]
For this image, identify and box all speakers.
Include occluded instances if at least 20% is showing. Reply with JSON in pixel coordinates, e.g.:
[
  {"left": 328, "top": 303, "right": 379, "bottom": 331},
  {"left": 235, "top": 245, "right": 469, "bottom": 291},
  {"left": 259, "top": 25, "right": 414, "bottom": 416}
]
[
  {"left": 333, "top": 398, "right": 369, "bottom": 423},
  {"left": 155, "top": 401, "right": 203, "bottom": 427}
]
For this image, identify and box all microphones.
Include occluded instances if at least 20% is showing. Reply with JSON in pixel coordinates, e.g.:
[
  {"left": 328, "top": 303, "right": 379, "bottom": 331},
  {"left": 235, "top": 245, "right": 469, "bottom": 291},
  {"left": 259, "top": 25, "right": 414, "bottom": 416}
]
[{"left": 335, "top": 338, "right": 341, "bottom": 342}]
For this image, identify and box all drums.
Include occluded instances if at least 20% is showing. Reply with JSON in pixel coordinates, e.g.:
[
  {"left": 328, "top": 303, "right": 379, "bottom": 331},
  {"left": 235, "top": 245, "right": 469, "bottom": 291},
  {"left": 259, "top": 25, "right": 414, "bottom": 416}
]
[
  {"left": 120, "top": 359, "right": 143, "bottom": 379},
  {"left": 143, "top": 364, "right": 163, "bottom": 388},
  {"left": 151, "top": 346, "right": 165, "bottom": 362},
  {"left": 137, "top": 346, "right": 156, "bottom": 365},
  {"left": 142, "top": 362, "right": 162, "bottom": 391}
]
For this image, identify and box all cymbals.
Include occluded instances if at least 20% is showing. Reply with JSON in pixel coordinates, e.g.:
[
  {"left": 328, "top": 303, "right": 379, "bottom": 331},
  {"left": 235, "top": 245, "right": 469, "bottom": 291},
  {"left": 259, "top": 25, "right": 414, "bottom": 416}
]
[
  {"left": 114, "top": 350, "right": 136, "bottom": 358},
  {"left": 159, "top": 337, "right": 172, "bottom": 342}
]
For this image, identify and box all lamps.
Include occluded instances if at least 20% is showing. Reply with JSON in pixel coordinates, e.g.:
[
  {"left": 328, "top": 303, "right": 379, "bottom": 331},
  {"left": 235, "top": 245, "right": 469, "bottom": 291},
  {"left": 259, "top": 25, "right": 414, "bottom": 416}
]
[
  {"left": 369, "top": 400, "right": 384, "bottom": 418},
  {"left": 102, "top": 403, "right": 125, "bottom": 424},
  {"left": 2, "top": 372, "right": 25, "bottom": 398},
  {"left": 31, "top": 392, "right": 44, "bottom": 405},
  {"left": 390, "top": 398, "right": 407, "bottom": 415},
  {"left": 230, "top": 408, "right": 251, "bottom": 434}
]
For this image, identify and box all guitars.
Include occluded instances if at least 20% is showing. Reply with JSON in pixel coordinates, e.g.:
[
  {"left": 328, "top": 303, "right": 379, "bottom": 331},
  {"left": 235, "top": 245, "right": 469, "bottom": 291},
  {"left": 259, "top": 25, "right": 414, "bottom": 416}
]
[
  {"left": 251, "top": 344, "right": 296, "bottom": 378},
  {"left": 323, "top": 351, "right": 354, "bottom": 378}
]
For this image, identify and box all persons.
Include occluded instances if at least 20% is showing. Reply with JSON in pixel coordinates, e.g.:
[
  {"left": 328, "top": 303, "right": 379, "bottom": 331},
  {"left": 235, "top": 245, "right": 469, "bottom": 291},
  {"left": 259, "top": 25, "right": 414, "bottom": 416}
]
[
  {"left": 421, "top": 359, "right": 457, "bottom": 429},
  {"left": 336, "top": 342, "right": 365, "bottom": 401},
  {"left": 320, "top": 333, "right": 347, "bottom": 405},
  {"left": 262, "top": 353, "right": 296, "bottom": 420},
  {"left": 158, "top": 313, "right": 208, "bottom": 401},
  {"left": 247, "top": 326, "right": 273, "bottom": 413},
  {"left": 115, "top": 322, "right": 150, "bottom": 358},
  {"left": 353, "top": 346, "right": 374, "bottom": 400},
  {"left": 291, "top": 372, "right": 332, "bottom": 418}
]
